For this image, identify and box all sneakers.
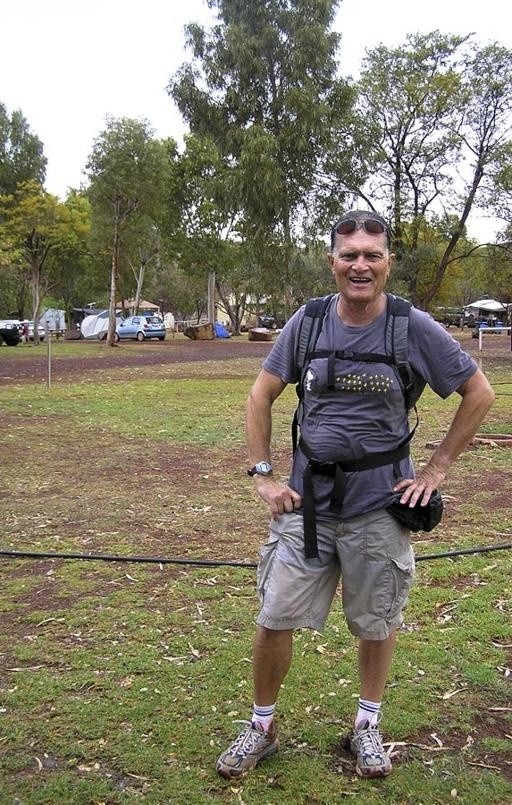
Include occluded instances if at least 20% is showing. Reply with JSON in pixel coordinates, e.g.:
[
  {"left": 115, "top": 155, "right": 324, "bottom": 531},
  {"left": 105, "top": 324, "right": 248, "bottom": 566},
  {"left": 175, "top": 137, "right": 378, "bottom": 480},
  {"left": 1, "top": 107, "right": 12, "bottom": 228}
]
[
  {"left": 216, "top": 720, "right": 279, "bottom": 779},
  {"left": 349, "top": 719, "right": 392, "bottom": 777}
]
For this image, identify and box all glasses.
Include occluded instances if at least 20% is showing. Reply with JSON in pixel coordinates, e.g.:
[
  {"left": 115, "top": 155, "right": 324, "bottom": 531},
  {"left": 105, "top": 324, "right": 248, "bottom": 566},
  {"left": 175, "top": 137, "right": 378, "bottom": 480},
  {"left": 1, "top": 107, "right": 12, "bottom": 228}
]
[{"left": 334, "top": 218, "right": 387, "bottom": 234}]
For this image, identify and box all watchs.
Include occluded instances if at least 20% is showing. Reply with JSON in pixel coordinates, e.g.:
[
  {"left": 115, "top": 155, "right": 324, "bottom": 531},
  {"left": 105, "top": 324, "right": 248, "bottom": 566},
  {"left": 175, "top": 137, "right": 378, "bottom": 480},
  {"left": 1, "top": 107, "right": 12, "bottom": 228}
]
[{"left": 246, "top": 461, "right": 272, "bottom": 477}]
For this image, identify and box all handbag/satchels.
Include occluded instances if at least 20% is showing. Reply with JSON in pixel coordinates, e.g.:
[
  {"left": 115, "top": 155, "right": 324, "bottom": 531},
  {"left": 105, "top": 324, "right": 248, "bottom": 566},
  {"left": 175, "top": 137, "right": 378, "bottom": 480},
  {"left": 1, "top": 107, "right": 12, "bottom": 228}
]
[{"left": 385, "top": 489, "right": 444, "bottom": 532}]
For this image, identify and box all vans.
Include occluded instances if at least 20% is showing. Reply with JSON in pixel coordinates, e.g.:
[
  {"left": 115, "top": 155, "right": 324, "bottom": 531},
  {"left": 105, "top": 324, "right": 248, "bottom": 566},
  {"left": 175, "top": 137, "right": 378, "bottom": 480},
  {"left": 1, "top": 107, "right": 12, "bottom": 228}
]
[{"left": 435, "top": 306, "right": 476, "bottom": 327}]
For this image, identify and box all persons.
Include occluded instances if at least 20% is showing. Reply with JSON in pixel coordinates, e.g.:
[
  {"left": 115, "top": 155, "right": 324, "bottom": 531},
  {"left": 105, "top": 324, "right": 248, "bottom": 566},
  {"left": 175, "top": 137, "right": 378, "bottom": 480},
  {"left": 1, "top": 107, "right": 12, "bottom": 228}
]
[{"left": 216, "top": 209, "right": 495, "bottom": 779}]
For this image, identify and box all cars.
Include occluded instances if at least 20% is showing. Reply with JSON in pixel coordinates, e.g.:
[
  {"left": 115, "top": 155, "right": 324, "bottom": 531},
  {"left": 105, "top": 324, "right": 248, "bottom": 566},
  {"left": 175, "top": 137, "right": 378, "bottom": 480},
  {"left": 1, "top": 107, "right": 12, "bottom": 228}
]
[
  {"left": 259, "top": 311, "right": 284, "bottom": 329},
  {"left": 114, "top": 316, "right": 166, "bottom": 343},
  {"left": 18, "top": 320, "right": 46, "bottom": 342}
]
